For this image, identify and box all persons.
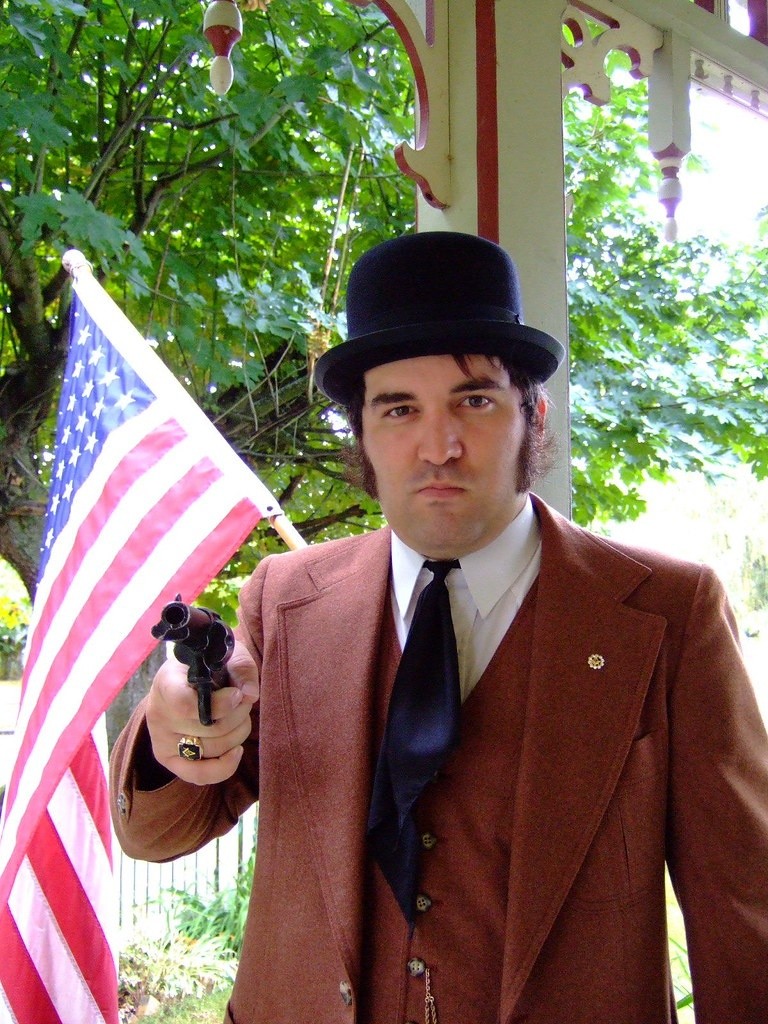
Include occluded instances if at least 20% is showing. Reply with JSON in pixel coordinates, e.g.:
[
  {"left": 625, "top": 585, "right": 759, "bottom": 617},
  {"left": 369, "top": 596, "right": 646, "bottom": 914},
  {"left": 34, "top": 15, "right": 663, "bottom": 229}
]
[{"left": 110, "top": 230, "right": 768, "bottom": 1024}]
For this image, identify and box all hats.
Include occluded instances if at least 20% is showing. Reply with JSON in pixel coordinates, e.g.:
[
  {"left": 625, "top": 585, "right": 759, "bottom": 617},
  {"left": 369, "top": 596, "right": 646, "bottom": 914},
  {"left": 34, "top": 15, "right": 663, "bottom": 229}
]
[{"left": 312, "top": 230, "right": 565, "bottom": 407}]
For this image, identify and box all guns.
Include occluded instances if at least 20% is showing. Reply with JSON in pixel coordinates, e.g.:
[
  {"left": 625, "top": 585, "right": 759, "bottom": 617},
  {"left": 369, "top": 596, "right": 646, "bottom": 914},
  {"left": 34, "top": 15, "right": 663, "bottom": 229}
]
[{"left": 150, "top": 593, "right": 236, "bottom": 727}]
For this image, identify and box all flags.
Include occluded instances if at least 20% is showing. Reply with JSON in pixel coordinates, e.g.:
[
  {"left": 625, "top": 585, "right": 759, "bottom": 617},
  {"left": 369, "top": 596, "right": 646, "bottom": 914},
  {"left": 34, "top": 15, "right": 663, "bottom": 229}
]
[{"left": 0, "top": 272, "right": 286, "bottom": 1024}]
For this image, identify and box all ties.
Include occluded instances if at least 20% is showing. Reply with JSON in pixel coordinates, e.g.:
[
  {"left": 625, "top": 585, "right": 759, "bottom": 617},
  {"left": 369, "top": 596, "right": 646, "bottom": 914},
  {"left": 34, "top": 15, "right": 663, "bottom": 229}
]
[{"left": 370, "top": 559, "right": 464, "bottom": 932}]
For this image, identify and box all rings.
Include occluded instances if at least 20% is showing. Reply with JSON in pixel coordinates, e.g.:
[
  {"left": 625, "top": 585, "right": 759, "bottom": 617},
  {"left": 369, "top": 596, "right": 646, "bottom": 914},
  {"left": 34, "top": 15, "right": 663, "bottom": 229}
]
[{"left": 177, "top": 736, "right": 204, "bottom": 761}]
